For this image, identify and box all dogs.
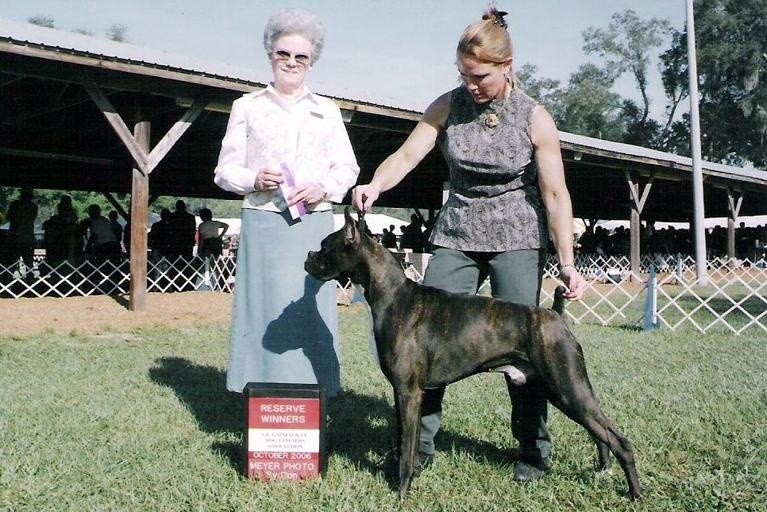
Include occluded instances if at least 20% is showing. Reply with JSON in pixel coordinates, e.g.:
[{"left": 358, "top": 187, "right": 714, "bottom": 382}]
[{"left": 303, "top": 205, "right": 643, "bottom": 503}]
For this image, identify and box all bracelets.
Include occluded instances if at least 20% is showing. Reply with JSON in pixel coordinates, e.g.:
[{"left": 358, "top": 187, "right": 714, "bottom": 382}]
[{"left": 563, "top": 265, "right": 578, "bottom": 271}]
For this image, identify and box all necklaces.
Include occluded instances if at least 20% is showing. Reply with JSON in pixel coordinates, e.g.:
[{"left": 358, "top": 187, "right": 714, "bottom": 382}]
[
  {"left": 274, "top": 85, "right": 302, "bottom": 100},
  {"left": 480, "top": 82, "right": 510, "bottom": 128}
]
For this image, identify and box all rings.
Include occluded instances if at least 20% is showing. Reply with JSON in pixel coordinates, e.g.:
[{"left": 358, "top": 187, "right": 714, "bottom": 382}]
[{"left": 305, "top": 196, "right": 310, "bottom": 202}]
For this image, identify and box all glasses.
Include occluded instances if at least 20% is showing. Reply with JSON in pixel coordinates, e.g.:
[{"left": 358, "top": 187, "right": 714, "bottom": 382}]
[{"left": 274, "top": 46, "right": 311, "bottom": 65}]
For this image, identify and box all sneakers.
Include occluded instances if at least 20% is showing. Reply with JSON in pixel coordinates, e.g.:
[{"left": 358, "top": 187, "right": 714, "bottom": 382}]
[
  {"left": 512, "top": 454, "right": 549, "bottom": 481},
  {"left": 394, "top": 451, "right": 435, "bottom": 476}
]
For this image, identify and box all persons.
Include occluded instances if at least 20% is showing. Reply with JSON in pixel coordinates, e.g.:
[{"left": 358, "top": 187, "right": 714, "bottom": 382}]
[
  {"left": 573, "top": 224, "right": 767, "bottom": 265},
  {"left": 352, "top": 1, "right": 586, "bottom": 481},
  {"left": 7, "top": 186, "right": 229, "bottom": 282},
  {"left": 213, "top": 9, "right": 361, "bottom": 398},
  {"left": 382, "top": 213, "right": 436, "bottom": 253}
]
